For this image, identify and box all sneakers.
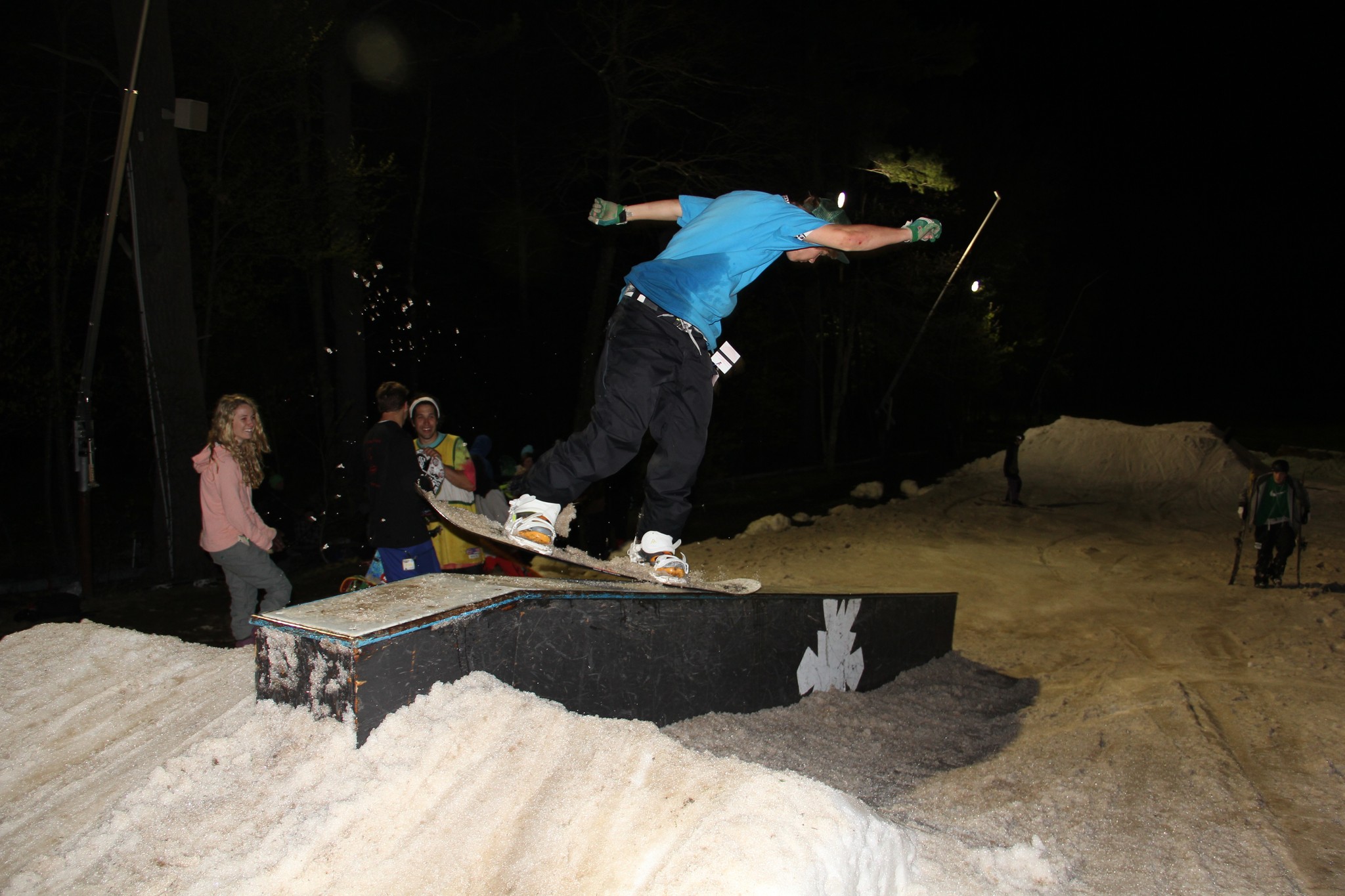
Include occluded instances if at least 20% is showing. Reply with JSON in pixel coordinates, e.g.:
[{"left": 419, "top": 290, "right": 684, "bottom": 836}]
[
  {"left": 512, "top": 492, "right": 553, "bottom": 544},
  {"left": 637, "top": 546, "right": 684, "bottom": 577}
]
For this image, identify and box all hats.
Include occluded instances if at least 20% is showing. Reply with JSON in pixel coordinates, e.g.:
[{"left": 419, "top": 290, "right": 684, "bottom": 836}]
[{"left": 810, "top": 196, "right": 851, "bottom": 264}]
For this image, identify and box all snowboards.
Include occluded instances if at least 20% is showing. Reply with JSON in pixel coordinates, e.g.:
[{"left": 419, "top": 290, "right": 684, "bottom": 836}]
[
  {"left": 347, "top": 447, "right": 445, "bottom": 594},
  {"left": 416, "top": 483, "right": 762, "bottom": 596}
]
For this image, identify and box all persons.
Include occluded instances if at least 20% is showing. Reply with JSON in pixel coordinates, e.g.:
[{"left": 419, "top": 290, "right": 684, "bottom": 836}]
[
  {"left": 409, "top": 397, "right": 486, "bottom": 575},
  {"left": 502, "top": 190, "right": 941, "bottom": 584},
  {"left": 363, "top": 381, "right": 441, "bottom": 583},
  {"left": 474, "top": 448, "right": 521, "bottom": 575},
  {"left": 1248, "top": 460, "right": 1308, "bottom": 588},
  {"left": 514, "top": 452, "right": 533, "bottom": 475},
  {"left": 191, "top": 394, "right": 293, "bottom": 647},
  {"left": 1003, "top": 434, "right": 1025, "bottom": 504}
]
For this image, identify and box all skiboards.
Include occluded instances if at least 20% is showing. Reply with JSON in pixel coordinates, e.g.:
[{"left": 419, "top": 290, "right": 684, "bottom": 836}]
[
  {"left": 974, "top": 498, "right": 1027, "bottom": 508},
  {"left": 1228, "top": 465, "right": 1257, "bottom": 586},
  {"left": 1296, "top": 471, "right": 1306, "bottom": 585}
]
